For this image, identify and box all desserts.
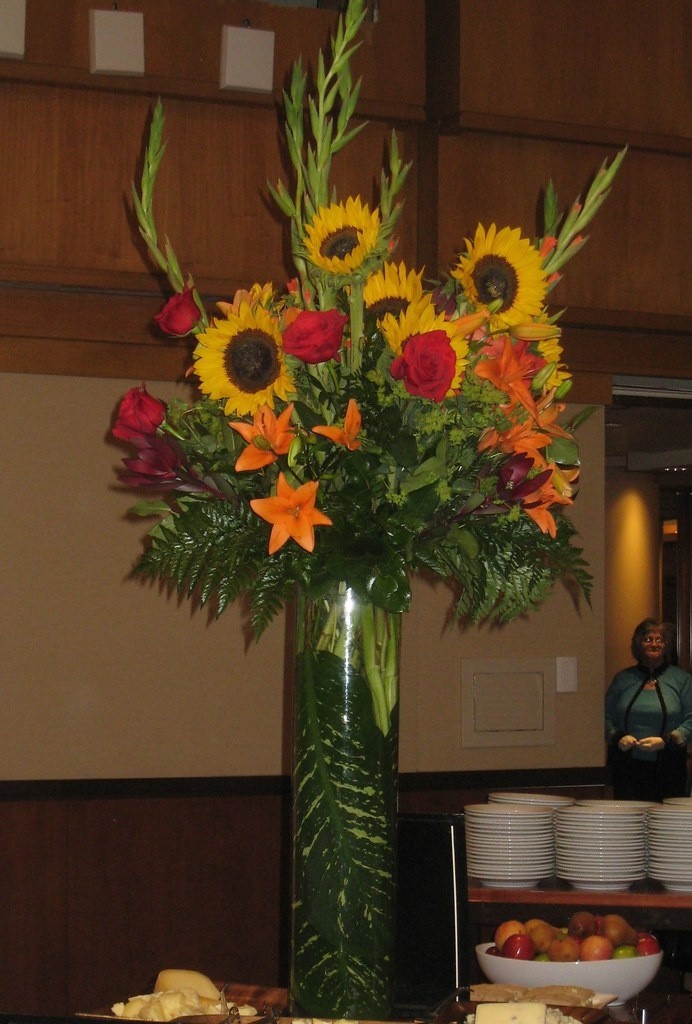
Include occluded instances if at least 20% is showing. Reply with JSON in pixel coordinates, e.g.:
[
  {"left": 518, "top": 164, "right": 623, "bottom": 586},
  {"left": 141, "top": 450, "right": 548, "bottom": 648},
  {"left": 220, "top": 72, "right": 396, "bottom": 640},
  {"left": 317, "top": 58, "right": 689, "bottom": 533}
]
[{"left": 109, "top": 970, "right": 256, "bottom": 1024}]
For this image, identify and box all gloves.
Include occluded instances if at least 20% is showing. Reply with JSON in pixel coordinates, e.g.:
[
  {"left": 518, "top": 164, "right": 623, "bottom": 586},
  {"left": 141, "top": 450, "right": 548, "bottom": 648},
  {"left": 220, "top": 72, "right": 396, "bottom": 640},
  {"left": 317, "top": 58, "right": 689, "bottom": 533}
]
[
  {"left": 618, "top": 736, "right": 638, "bottom": 750},
  {"left": 636, "top": 736, "right": 666, "bottom": 751}
]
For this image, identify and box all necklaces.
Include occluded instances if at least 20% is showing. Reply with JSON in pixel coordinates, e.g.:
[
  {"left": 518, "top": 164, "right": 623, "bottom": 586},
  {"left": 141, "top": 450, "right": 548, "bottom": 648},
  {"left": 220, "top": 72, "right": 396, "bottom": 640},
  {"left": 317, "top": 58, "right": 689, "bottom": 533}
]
[{"left": 646, "top": 678, "right": 658, "bottom": 686}]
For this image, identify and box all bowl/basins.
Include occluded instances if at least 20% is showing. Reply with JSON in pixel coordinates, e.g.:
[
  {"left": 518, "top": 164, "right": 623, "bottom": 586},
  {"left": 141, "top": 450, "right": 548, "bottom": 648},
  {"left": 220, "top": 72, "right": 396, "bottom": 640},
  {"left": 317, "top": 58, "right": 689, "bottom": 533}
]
[{"left": 472, "top": 941, "right": 664, "bottom": 1007}]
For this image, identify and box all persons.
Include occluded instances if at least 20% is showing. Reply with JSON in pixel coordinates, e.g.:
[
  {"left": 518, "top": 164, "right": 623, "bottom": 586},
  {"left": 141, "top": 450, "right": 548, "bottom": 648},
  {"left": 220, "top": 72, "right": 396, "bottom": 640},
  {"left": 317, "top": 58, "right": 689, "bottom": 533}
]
[{"left": 604, "top": 617, "right": 692, "bottom": 803}]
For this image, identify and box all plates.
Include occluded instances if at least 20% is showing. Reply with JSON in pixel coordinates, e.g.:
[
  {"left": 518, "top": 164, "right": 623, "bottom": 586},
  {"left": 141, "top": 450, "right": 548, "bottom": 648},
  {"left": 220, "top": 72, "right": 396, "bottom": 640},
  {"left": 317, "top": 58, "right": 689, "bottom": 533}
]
[{"left": 465, "top": 789, "right": 691, "bottom": 893}]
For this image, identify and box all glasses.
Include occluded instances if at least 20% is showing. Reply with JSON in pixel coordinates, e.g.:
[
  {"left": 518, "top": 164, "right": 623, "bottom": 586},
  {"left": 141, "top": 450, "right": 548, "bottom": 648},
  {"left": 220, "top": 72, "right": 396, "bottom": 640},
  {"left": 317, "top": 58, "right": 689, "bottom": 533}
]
[{"left": 640, "top": 637, "right": 666, "bottom": 644}]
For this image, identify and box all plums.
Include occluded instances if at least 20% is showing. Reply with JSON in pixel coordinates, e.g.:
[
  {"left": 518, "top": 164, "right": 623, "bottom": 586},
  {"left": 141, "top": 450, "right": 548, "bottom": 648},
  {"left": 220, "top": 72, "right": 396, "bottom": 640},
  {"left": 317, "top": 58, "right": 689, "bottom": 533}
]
[{"left": 485, "top": 911, "right": 661, "bottom": 963}]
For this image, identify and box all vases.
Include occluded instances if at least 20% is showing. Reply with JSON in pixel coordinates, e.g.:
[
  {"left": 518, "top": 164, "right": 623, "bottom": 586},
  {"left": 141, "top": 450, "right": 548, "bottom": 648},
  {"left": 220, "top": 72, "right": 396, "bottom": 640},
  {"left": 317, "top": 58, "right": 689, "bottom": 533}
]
[{"left": 289, "top": 544, "right": 412, "bottom": 1018}]
